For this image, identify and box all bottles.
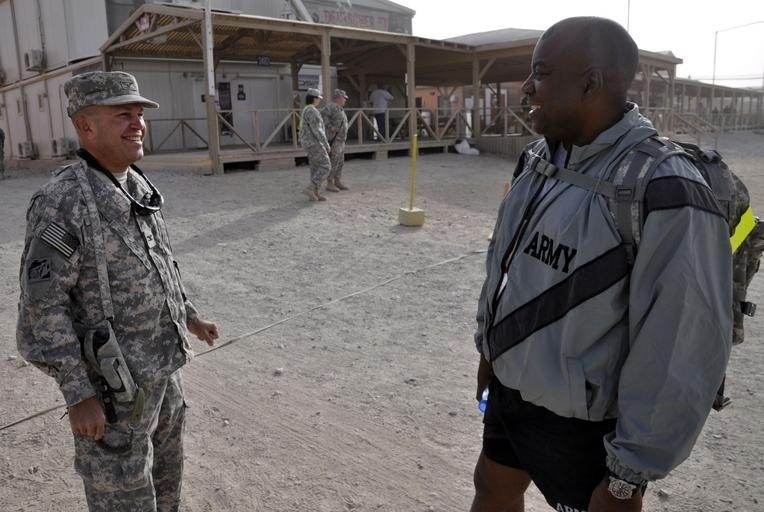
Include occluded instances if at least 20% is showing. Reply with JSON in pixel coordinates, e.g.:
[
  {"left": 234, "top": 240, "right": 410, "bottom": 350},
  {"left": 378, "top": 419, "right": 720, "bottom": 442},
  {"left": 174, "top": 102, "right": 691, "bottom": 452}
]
[{"left": 477, "top": 385, "right": 490, "bottom": 412}]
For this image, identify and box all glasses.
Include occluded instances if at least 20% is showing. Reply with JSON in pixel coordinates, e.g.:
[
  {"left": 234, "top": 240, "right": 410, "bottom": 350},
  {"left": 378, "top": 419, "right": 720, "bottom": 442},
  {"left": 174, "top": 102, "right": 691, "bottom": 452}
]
[{"left": 120, "top": 174, "right": 164, "bottom": 216}]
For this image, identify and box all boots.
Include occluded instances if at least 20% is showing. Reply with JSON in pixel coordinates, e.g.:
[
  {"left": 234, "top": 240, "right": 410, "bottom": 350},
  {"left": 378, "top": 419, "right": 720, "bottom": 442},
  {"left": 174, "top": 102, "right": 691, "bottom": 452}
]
[
  {"left": 335, "top": 177, "right": 348, "bottom": 190},
  {"left": 302, "top": 183, "right": 326, "bottom": 201},
  {"left": 328, "top": 179, "right": 339, "bottom": 192}
]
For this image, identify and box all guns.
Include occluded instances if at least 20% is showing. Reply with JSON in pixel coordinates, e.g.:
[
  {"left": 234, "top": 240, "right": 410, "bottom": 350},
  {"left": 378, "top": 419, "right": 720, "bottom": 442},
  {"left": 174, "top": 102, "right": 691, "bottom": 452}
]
[{"left": 76, "top": 332, "right": 136, "bottom": 404}]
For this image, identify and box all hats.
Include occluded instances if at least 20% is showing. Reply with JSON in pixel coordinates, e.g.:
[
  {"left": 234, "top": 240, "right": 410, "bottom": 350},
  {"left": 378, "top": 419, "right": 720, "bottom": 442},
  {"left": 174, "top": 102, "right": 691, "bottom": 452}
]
[
  {"left": 64, "top": 70, "right": 159, "bottom": 119},
  {"left": 334, "top": 89, "right": 349, "bottom": 99},
  {"left": 308, "top": 88, "right": 323, "bottom": 98}
]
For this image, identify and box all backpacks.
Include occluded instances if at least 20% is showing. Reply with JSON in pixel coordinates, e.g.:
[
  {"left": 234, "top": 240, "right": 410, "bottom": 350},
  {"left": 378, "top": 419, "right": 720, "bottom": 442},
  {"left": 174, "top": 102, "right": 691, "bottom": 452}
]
[{"left": 524, "top": 137, "right": 763, "bottom": 411}]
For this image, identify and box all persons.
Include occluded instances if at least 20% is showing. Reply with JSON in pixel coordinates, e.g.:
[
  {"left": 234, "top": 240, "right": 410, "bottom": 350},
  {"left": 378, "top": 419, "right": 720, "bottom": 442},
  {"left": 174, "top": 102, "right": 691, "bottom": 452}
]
[
  {"left": 519, "top": 96, "right": 528, "bottom": 136},
  {"left": 11, "top": 70, "right": 218, "bottom": 511},
  {"left": 466, "top": 15, "right": 764, "bottom": 511},
  {"left": 319, "top": 88, "right": 350, "bottom": 191},
  {"left": 299, "top": 89, "right": 328, "bottom": 202},
  {"left": 368, "top": 80, "right": 393, "bottom": 140}
]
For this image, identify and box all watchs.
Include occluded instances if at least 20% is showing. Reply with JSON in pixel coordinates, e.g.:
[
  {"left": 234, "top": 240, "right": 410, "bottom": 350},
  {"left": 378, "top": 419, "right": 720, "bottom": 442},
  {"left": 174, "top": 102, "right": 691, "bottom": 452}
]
[{"left": 599, "top": 467, "right": 644, "bottom": 500}]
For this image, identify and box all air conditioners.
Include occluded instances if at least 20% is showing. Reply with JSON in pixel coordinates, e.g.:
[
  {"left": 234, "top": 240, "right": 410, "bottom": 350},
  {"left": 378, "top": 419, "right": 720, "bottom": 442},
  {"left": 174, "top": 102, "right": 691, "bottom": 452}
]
[
  {"left": 15, "top": 140, "right": 32, "bottom": 157},
  {"left": 48, "top": 138, "right": 69, "bottom": 156},
  {"left": 23, "top": 47, "right": 42, "bottom": 71}
]
